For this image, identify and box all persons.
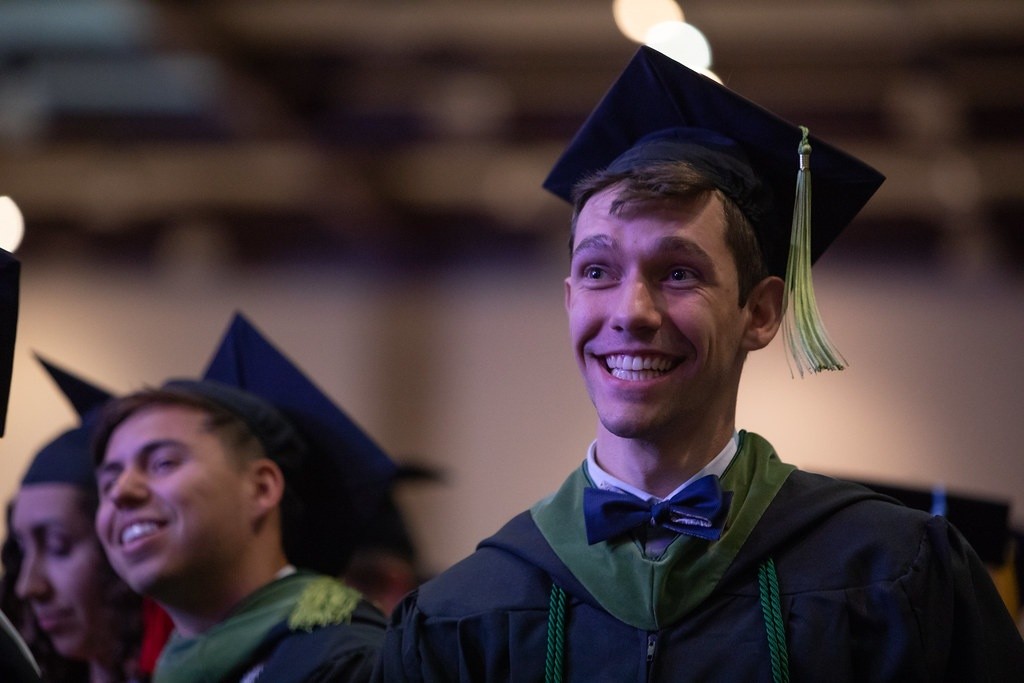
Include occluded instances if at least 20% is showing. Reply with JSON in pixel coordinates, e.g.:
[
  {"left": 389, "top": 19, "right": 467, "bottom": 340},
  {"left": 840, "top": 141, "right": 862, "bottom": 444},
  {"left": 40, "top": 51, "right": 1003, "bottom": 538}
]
[
  {"left": 371, "top": 146, "right": 1023, "bottom": 682},
  {"left": 10, "top": 428, "right": 178, "bottom": 682},
  {"left": 88, "top": 382, "right": 384, "bottom": 683}
]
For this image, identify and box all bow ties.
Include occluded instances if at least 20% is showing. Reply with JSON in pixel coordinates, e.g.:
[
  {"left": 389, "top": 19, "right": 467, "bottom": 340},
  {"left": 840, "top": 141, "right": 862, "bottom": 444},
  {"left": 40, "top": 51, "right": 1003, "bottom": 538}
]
[{"left": 584, "top": 474, "right": 733, "bottom": 545}]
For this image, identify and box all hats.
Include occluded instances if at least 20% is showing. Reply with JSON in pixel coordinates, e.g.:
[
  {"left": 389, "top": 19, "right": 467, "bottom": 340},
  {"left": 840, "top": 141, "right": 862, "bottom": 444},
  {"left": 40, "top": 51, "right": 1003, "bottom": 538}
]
[
  {"left": 541, "top": 44, "right": 886, "bottom": 380},
  {"left": 203, "top": 312, "right": 402, "bottom": 511},
  {"left": 20, "top": 351, "right": 123, "bottom": 486}
]
